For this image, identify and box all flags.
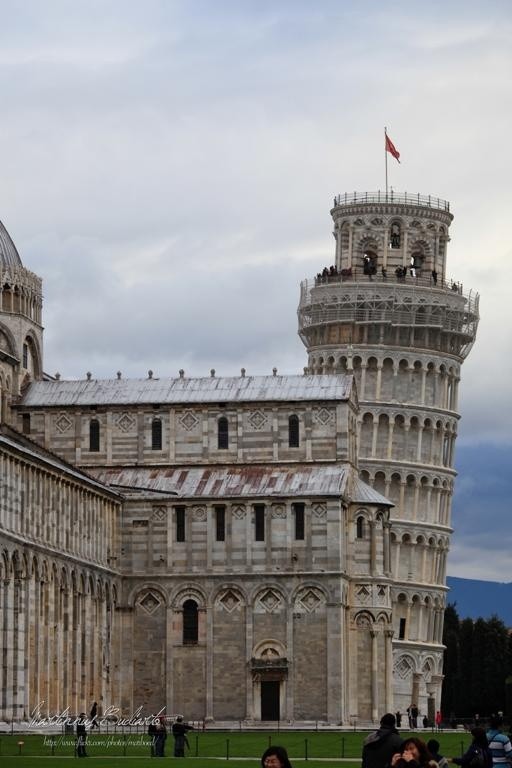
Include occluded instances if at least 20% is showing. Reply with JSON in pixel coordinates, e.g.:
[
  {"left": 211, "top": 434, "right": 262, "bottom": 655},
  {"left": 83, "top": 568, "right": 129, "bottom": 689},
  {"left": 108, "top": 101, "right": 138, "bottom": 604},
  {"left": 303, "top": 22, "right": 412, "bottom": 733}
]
[{"left": 385, "top": 132, "right": 401, "bottom": 163}]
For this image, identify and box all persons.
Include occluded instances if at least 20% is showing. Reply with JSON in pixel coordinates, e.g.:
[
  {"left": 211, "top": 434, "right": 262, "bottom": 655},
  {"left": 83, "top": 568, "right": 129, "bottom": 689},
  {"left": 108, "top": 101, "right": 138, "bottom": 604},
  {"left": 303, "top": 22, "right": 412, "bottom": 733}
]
[
  {"left": 315, "top": 254, "right": 407, "bottom": 286},
  {"left": 261, "top": 746, "right": 292, "bottom": 768},
  {"left": 148, "top": 714, "right": 194, "bottom": 757},
  {"left": 90, "top": 702, "right": 99, "bottom": 729},
  {"left": 432, "top": 270, "right": 437, "bottom": 286},
  {"left": 77, "top": 713, "right": 89, "bottom": 757},
  {"left": 361, "top": 704, "right": 512, "bottom": 768}
]
